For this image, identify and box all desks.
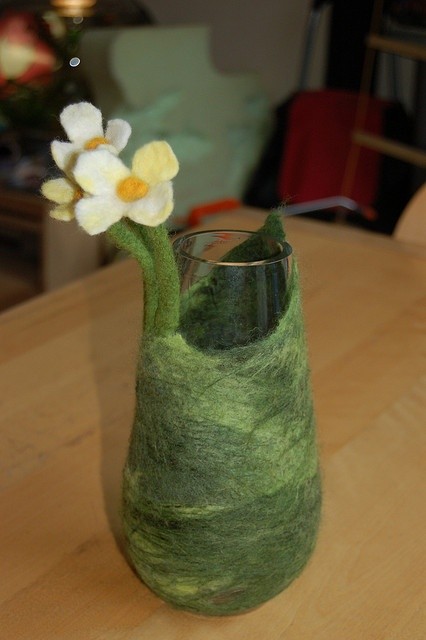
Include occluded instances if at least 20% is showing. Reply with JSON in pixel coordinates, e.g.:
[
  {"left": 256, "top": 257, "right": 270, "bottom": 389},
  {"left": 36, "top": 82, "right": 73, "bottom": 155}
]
[{"left": 1, "top": 208, "right": 425, "bottom": 640}]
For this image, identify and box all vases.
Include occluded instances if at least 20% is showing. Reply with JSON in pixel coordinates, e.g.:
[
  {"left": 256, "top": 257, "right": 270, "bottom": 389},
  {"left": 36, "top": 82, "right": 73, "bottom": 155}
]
[{"left": 121, "top": 228, "right": 322, "bottom": 619}]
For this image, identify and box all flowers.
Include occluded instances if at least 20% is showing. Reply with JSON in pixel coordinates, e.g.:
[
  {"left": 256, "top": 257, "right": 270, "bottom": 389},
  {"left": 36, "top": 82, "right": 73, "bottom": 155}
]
[{"left": 37, "top": 101, "right": 181, "bottom": 340}]
